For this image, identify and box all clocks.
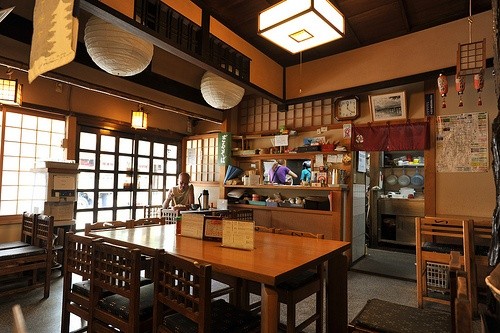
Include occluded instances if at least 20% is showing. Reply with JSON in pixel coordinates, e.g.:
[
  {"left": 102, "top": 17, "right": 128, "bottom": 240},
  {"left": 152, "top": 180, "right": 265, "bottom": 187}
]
[{"left": 334, "top": 95, "right": 361, "bottom": 121}]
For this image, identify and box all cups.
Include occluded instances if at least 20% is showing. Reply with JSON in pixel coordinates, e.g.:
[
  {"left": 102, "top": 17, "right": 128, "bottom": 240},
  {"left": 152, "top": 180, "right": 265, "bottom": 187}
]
[
  {"left": 296, "top": 197, "right": 301, "bottom": 204},
  {"left": 289, "top": 198, "right": 295, "bottom": 203}
]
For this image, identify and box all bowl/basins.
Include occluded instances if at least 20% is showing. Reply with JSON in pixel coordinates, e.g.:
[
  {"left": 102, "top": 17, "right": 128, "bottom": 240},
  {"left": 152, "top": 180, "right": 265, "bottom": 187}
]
[{"left": 190, "top": 204, "right": 200, "bottom": 210}]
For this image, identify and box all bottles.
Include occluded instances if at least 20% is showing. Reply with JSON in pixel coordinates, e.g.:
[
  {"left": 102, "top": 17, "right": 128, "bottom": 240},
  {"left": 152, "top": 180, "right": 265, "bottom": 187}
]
[
  {"left": 332, "top": 168, "right": 346, "bottom": 185},
  {"left": 176, "top": 216, "right": 181, "bottom": 236}
]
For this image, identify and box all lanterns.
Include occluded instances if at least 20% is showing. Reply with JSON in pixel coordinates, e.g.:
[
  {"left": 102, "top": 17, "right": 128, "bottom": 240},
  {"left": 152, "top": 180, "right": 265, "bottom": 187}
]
[
  {"left": 201, "top": 70, "right": 245, "bottom": 110},
  {"left": 84, "top": 15, "right": 154, "bottom": 75}
]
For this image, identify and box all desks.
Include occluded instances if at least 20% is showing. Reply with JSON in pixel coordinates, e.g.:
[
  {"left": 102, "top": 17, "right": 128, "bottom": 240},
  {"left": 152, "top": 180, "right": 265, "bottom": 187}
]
[{"left": 89, "top": 222, "right": 352, "bottom": 333}]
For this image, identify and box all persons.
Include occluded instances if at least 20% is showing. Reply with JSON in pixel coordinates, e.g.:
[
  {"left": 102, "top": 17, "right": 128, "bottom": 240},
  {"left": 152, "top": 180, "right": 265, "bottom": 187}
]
[
  {"left": 162, "top": 172, "right": 195, "bottom": 209},
  {"left": 300, "top": 161, "right": 311, "bottom": 184},
  {"left": 268, "top": 158, "right": 298, "bottom": 185}
]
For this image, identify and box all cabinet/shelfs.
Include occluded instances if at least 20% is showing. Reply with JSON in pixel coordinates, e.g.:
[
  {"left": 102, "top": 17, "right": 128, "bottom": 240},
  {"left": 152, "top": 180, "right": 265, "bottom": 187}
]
[
  {"left": 27, "top": 219, "right": 77, "bottom": 277},
  {"left": 223, "top": 134, "right": 351, "bottom": 243},
  {"left": 415, "top": 213, "right": 492, "bottom": 309}
]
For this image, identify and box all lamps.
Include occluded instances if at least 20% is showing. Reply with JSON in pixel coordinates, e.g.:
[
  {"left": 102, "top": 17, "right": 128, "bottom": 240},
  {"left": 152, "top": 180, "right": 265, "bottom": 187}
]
[
  {"left": 257, "top": 0, "right": 345, "bottom": 53},
  {"left": 0, "top": 69, "right": 24, "bottom": 108},
  {"left": 457, "top": 0, "right": 487, "bottom": 78},
  {"left": 130, "top": 104, "right": 148, "bottom": 131}
]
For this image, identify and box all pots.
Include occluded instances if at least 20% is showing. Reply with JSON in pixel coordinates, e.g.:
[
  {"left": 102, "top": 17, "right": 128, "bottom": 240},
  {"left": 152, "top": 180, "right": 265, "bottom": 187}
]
[
  {"left": 385, "top": 168, "right": 397, "bottom": 186},
  {"left": 411, "top": 166, "right": 424, "bottom": 186},
  {"left": 397, "top": 167, "right": 411, "bottom": 187}
]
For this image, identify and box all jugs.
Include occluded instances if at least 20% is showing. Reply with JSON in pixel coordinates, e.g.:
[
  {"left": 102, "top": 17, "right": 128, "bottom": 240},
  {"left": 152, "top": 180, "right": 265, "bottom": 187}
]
[{"left": 198, "top": 190, "right": 208, "bottom": 210}]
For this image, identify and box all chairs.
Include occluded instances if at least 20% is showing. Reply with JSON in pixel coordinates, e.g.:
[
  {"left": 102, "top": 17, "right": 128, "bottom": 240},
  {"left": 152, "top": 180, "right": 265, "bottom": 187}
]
[
  {"left": 0, "top": 211, "right": 324, "bottom": 333},
  {"left": 350, "top": 220, "right": 500, "bottom": 333}
]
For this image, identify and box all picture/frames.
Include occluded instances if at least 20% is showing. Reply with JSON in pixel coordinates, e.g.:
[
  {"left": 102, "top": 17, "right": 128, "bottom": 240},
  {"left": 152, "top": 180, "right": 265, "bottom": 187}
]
[{"left": 369, "top": 88, "right": 408, "bottom": 124}]
